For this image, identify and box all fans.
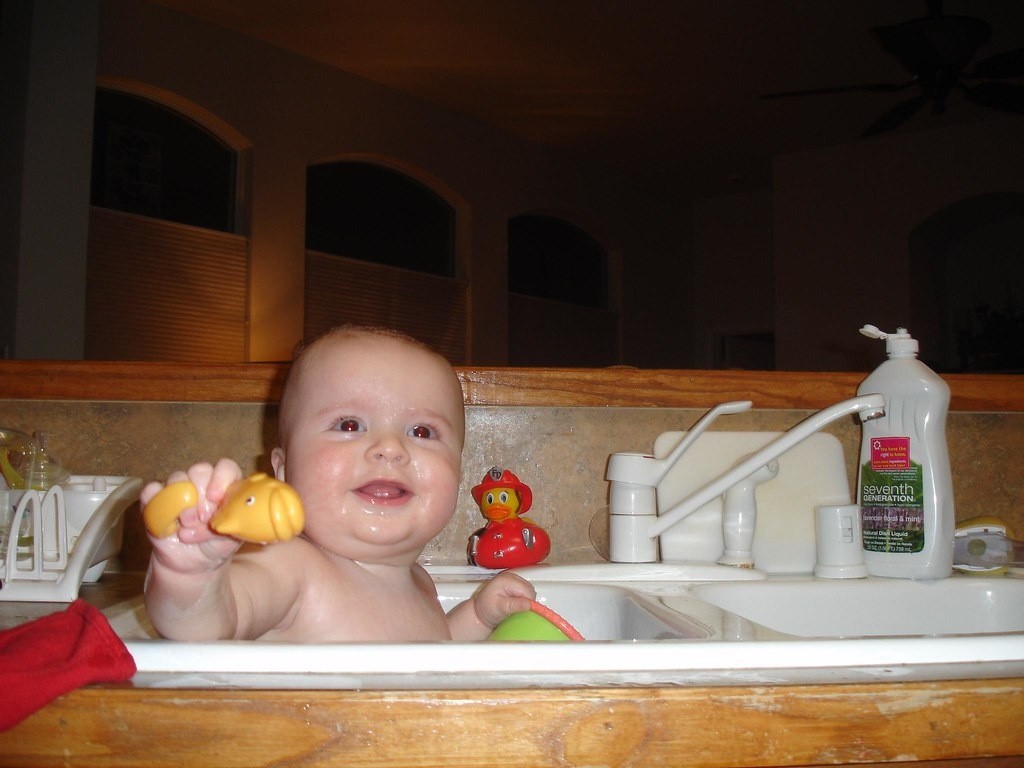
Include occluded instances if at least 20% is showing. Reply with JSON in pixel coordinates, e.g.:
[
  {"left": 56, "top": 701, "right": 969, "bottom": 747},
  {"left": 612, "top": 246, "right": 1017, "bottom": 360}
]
[{"left": 758, "top": 0, "right": 1024, "bottom": 138}]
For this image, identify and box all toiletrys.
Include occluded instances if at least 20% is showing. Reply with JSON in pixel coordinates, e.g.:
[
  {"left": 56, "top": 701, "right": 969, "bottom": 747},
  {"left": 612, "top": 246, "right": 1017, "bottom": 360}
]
[{"left": 854, "top": 322, "right": 957, "bottom": 582}]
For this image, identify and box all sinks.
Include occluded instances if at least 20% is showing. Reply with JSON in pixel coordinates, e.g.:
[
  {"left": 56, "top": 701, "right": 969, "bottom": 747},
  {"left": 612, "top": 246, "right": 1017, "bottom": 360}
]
[
  {"left": 99, "top": 564, "right": 712, "bottom": 690},
  {"left": 685, "top": 581, "right": 1024, "bottom": 688}
]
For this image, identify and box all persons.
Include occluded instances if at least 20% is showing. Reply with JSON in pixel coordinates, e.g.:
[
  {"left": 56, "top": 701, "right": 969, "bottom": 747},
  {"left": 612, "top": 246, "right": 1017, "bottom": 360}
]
[{"left": 141, "top": 322, "right": 534, "bottom": 642}]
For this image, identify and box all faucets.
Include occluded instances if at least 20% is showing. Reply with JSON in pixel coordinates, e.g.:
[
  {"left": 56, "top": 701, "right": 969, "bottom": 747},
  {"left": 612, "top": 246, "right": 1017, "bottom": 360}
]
[{"left": 601, "top": 390, "right": 889, "bottom": 566}]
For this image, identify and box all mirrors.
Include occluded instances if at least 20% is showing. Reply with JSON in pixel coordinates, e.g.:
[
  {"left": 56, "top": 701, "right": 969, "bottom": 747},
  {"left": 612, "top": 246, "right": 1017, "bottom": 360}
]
[{"left": 1, "top": 0, "right": 1024, "bottom": 415}]
